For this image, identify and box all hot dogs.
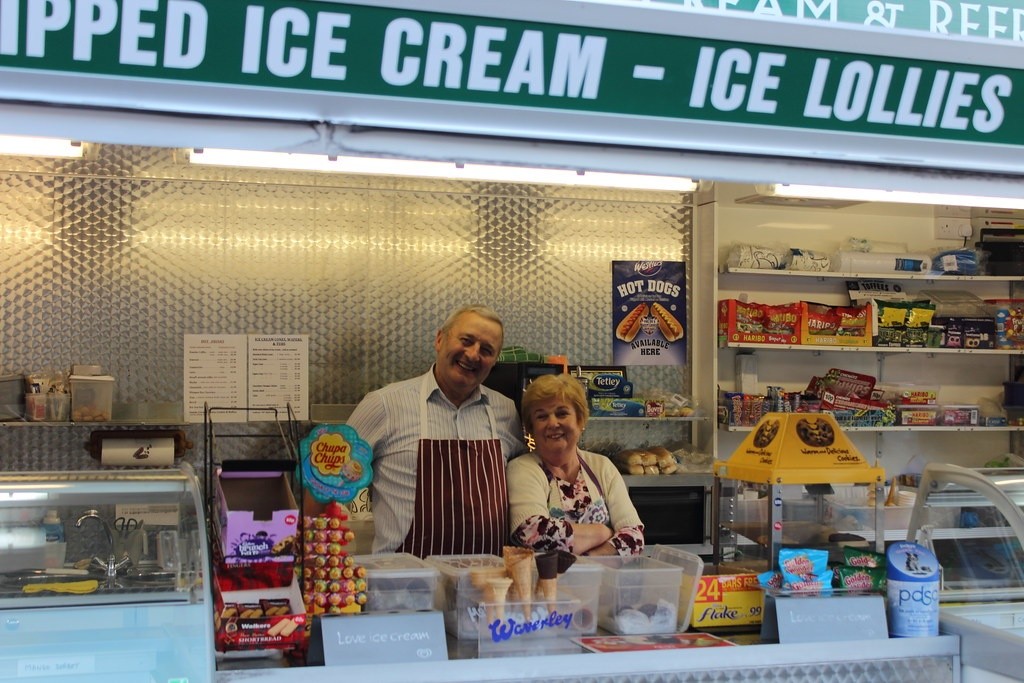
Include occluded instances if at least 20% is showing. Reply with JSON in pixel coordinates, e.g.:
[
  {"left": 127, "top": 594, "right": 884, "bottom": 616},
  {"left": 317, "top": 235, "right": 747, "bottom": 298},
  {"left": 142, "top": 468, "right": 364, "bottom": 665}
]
[
  {"left": 651, "top": 302, "right": 683, "bottom": 342},
  {"left": 616, "top": 304, "right": 649, "bottom": 342}
]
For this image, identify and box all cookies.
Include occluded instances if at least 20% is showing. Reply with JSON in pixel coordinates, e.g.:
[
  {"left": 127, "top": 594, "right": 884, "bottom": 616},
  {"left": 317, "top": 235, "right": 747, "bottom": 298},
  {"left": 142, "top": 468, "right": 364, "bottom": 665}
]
[
  {"left": 796, "top": 418, "right": 834, "bottom": 446},
  {"left": 753, "top": 421, "right": 780, "bottom": 447}
]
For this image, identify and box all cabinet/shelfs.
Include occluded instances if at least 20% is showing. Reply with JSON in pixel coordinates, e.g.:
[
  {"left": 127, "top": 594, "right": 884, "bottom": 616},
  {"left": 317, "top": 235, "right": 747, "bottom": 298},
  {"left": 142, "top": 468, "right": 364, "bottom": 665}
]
[{"left": 691, "top": 178, "right": 1024, "bottom": 471}]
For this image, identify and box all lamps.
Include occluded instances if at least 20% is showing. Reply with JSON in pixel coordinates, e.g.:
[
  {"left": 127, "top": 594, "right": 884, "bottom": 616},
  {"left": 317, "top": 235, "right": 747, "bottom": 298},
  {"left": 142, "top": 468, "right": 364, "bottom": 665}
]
[
  {"left": 756, "top": 183, "right": 1024, "bottom": 211},
  {"left": 188, "top": 148, "right": 701, "bottom": 193}
]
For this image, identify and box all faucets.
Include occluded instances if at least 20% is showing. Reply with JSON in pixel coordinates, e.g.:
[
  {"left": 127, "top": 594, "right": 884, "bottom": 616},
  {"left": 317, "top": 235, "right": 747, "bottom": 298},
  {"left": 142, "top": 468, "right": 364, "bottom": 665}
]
[{"left": 75, "top": 508, "right": 116, "bottom": 555}]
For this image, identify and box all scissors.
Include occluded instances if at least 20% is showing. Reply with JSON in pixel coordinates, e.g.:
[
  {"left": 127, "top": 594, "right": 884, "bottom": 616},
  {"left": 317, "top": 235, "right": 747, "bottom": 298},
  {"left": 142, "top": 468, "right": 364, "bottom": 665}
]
[{"left": 113, "top": 516, "right": 138, "bottom": 552}]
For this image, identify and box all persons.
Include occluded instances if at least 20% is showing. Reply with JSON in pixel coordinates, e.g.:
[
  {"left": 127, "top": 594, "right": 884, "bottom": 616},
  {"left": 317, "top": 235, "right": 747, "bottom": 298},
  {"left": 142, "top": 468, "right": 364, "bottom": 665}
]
[
  {"left": 506, "top": 373, "right": 643, "bottom": 567},
  {"left": 301, "top": 306, "right": 530, "bottom": 557}
]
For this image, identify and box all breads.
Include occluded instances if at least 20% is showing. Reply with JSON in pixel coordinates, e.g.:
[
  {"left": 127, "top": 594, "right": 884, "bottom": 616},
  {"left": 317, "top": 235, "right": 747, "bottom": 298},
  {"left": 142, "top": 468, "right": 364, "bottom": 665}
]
[{"left": 615, "top": 446, "right": 676, "bottom": 475}]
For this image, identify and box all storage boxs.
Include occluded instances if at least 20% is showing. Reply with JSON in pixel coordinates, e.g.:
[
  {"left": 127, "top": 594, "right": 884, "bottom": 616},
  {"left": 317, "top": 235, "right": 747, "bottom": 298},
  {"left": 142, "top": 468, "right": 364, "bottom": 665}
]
[
  {"left": 735, "top": 484, "right": 960, "bottom": 532},
  {"left": 352, "top": 542, "right": 767, "bottom": 641},
  {"left": 1001, "top": 380, "right": 1024, "bottom": 425},
  {"left": 0, "top": 373, "right": 116, "bottom": 422},
  {"left": 213, "top": 467, "right": 306, "bottom": 650},
  {"left": 717, "top": 368, "right": 981, "bottom": 428},
  {"left": 719, "top": 290, "right": 1024, "bottom": 350}
]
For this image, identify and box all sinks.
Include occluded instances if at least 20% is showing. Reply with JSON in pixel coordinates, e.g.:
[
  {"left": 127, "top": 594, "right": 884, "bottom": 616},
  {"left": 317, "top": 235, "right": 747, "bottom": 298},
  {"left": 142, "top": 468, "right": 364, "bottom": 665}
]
[
  {"left": 0, "top": 570, "right": 124, "bottom": 595},
  {"left": 120, "top": 572, "right": 191, "bottom": 586}
]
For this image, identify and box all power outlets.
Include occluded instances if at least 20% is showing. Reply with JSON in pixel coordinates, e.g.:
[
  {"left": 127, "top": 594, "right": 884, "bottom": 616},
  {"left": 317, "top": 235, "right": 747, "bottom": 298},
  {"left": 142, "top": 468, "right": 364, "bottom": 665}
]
[{"left": 934, "top": 217, "right": 971, "bottom": 241}]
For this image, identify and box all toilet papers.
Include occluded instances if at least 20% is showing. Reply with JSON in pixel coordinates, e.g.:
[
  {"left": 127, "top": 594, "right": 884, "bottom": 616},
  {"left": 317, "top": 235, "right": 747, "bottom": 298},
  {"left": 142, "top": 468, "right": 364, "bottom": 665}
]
[{"left": 100, "top": 437, "right": 175, "bottom": 467}]
